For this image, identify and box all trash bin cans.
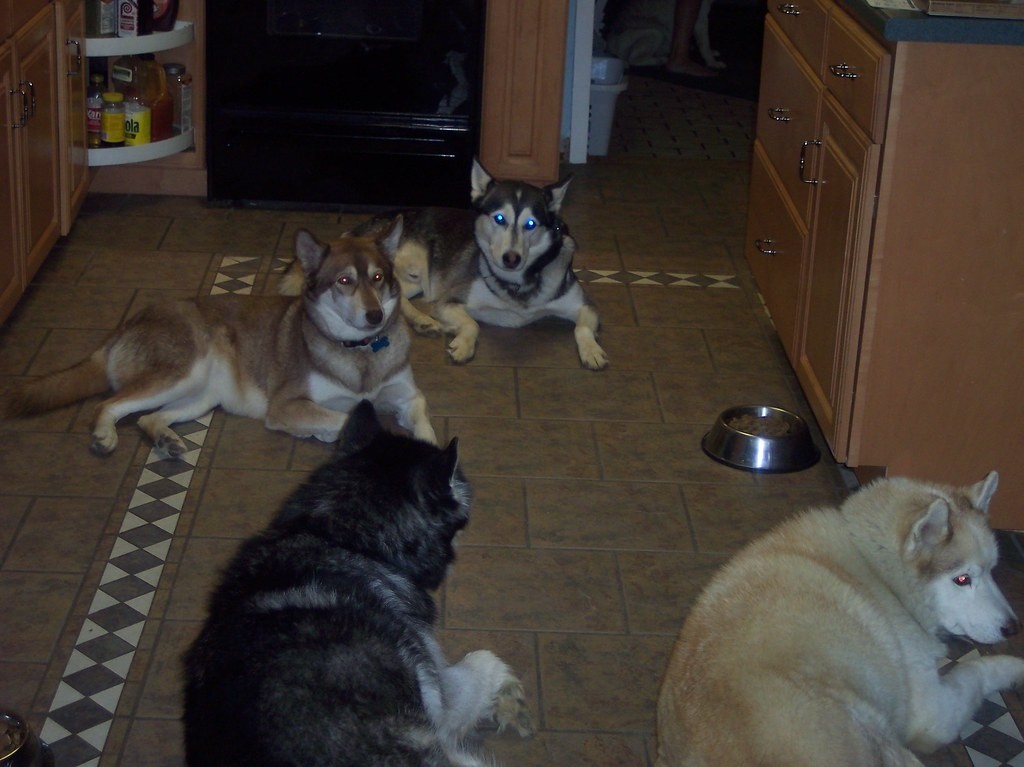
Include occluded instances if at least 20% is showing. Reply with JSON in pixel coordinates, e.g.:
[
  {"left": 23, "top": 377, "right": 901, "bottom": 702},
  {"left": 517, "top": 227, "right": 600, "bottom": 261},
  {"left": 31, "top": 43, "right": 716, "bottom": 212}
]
[{"left": 588, "top": 57, "right": 630, "bottom": 155}]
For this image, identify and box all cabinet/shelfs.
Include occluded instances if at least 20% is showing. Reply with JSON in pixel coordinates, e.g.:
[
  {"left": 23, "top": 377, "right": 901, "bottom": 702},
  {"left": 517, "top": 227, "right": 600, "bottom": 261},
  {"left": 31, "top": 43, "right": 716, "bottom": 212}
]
[
  {"left": 85, "top": 21, "right": 195, "bottom": 166},
  {"left": 745, "top": 0, "right": 1024, "bottom": 533},
  {"left": 0, "top": 0, "right": 88, "bottom": 321}
]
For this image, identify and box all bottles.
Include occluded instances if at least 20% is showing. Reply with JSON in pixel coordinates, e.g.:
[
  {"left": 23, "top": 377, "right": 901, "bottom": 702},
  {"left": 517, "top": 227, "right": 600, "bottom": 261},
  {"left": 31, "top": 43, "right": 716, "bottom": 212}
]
[
  {"left": 86, "top": 75, "right": 106, "bottom": 148},
  {"left": 82, "top": 0, "right": 179, "bottom": 38},
  {"left": 112, "top": 54, "right": 193, "bottom": 148}
]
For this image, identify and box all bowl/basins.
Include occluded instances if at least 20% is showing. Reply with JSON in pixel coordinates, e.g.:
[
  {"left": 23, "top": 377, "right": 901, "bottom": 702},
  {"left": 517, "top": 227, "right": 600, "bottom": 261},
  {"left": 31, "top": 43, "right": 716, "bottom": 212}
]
[{"left": 703, "top": 403, "right": 821, "bottom": 474}]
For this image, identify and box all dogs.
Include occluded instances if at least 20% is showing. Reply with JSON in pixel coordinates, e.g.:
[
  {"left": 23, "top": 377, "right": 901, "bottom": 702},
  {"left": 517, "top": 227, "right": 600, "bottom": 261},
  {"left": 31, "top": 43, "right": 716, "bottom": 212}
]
[
  {"left": 178, "top": 399, "right": 526, "bottom": 767},
  {"left": 0, "top": 160, "right": 608, "bottom": 460},
  {"left": 654, "top": 468, "right": 1024, "bottom": 767}
]
